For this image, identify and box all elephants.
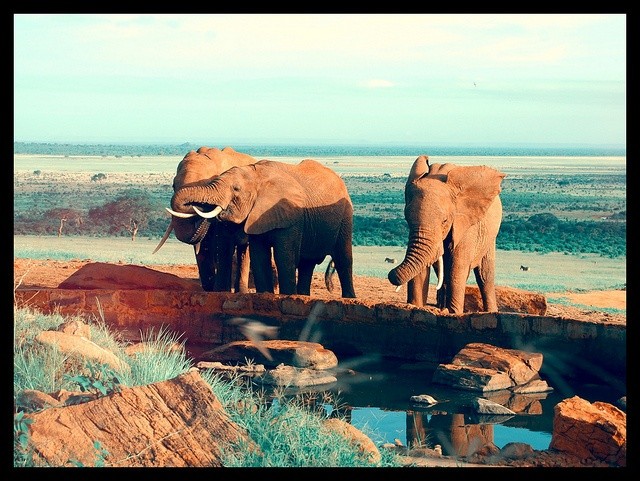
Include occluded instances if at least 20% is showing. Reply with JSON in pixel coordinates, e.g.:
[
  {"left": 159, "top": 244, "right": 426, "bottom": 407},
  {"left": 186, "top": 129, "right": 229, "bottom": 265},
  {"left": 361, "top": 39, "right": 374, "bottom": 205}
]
[
  {"left": 387, "top": 155, "right": 503, "bottom": 316},
  {"left": 155, "top": 146, "right": 255, "bottom": 293},
  {"left": 164, "top": 161, "right": 358, "bottom": 298}
]
